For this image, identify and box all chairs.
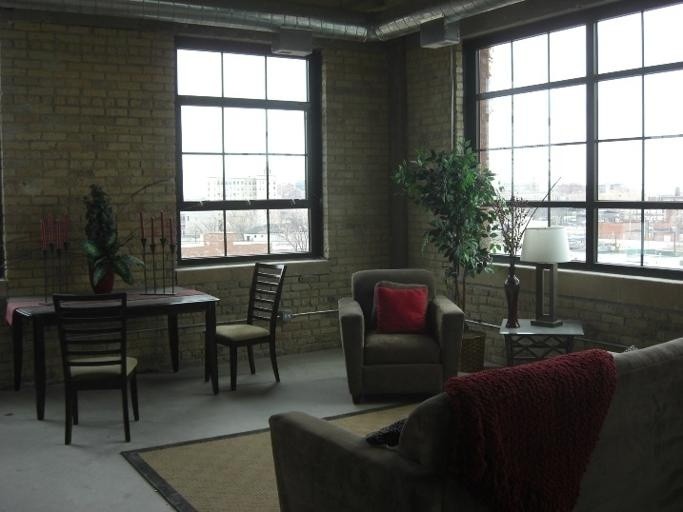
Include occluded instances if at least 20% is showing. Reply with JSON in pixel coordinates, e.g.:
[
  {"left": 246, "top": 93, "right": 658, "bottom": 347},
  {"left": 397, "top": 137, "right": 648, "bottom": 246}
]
[
  {"left": 48, "top": 293, "right": 140, "bottom": 445},
  {"left": 336, "top": 268, "right": 467, "bottom": 401},
  {"left": 205, "top": 262, "right": 288, "bottom": 390}
]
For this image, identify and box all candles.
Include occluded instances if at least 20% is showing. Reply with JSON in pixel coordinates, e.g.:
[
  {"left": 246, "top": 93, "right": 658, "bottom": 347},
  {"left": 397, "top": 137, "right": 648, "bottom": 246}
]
[
  {"left": 139, "top": 209, "right": 173, "bottom": 245},
  {"left": 39, "top": 207, "right": 68, "bottom": 250}
]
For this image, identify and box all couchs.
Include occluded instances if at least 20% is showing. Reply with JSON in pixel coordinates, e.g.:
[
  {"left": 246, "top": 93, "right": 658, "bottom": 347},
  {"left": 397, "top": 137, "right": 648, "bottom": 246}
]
[{"left": 269, "top": 334, "right": 682, "bottom": 512}]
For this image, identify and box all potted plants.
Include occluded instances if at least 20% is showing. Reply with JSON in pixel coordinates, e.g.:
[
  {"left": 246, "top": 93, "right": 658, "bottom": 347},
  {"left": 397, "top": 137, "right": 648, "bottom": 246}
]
[
  {"left": 79, "top": 185, "right": 147, "bottom": 295},
  {"left": 483, "top": 177, "right": 561, "bottom": 328},
  {"left": 396, "top": 138, "right": 498, "bottom": 372}
]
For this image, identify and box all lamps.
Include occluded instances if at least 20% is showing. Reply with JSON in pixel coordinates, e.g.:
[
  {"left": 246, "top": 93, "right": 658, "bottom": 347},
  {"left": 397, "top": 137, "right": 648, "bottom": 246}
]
[{"left": 520, "top": 225, "right": 572, "bottom": 328}]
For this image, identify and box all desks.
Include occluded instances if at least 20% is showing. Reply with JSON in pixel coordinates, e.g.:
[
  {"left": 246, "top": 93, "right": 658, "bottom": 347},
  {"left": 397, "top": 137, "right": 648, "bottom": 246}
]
[{"left": 5, "top": 284, "right": 221, "bottom": 421}]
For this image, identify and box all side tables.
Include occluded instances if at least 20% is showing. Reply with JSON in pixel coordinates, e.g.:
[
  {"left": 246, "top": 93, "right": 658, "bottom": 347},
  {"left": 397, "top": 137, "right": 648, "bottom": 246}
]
[{"left": 498, "top": 316, "right": 585, "bottom": 369}]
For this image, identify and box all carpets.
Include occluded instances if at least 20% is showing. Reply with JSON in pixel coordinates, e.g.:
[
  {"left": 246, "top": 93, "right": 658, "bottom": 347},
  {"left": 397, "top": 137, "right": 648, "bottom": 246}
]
[{"left": 121, "top": 395, "right": 434, "bottom": 510}]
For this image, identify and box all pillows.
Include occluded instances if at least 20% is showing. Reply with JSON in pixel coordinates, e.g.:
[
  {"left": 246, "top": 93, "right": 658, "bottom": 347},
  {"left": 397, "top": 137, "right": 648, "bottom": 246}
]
[{"left": 369, "top": 279, "right": 432, "bottom": 335}]
